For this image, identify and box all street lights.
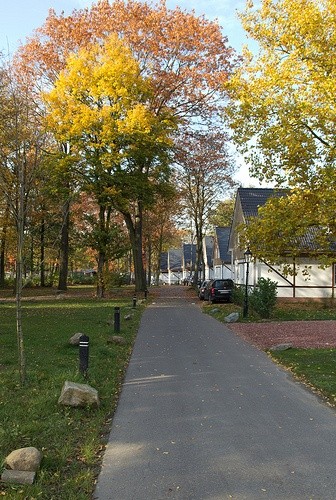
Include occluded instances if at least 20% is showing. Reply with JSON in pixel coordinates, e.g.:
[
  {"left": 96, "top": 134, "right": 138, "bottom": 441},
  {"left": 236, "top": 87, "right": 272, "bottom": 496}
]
[{"left": 241, "top": 247, "right": 253, "bottom": 319}]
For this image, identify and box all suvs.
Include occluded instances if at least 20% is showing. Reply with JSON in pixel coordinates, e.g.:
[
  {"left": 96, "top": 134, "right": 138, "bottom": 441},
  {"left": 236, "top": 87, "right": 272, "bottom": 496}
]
[{"left": 203, "top": 279, "right": 235, "bottom": 303}]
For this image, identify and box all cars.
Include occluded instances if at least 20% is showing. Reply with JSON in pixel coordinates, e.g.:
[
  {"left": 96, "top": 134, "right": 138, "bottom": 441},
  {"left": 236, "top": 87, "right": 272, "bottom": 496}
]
[{"left": 198, "top": 279, "right": 211, "bottom": 300}]
